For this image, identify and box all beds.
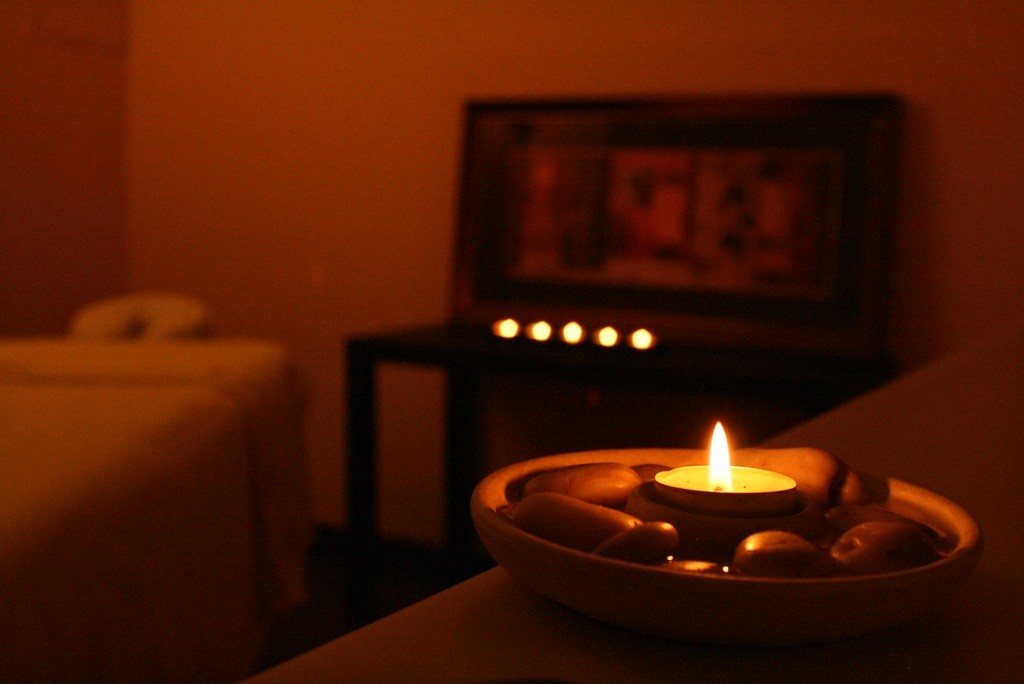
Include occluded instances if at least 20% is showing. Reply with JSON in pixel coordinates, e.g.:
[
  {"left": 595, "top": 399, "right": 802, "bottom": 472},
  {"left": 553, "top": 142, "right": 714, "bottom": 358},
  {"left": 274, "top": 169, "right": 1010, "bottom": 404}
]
[{"left": 0, "top": 338, "right": 313, "bottom": 684}]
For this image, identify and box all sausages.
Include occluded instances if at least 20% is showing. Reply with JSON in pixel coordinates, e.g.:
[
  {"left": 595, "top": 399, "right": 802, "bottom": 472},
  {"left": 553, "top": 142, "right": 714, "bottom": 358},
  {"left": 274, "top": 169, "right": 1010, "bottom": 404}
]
[
  {"left": 592, "top": 520, "right": 678, "bottom": 565},
  {"left": 524, "top": 464, "right": 640, "bottom": 505},
  {"left": 512, "top": 494, "right": 641, "bottom": 549}
]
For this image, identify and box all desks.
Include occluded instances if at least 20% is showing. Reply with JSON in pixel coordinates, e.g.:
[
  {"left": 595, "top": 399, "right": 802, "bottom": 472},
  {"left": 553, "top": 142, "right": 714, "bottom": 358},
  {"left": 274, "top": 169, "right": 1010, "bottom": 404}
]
[
  {"left": 345, "top": 325, "right": 919, "bottom": 633},
  {"left": 235, "top": 316, "right": 1024, "bottom": 684}
]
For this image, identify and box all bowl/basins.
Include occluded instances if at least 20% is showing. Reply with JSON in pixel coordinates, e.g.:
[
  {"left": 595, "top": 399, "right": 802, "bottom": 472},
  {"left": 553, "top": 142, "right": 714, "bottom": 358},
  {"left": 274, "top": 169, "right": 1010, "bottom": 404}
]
[{"left": 469, "top": 448, "right": 982, "bottom": 647}]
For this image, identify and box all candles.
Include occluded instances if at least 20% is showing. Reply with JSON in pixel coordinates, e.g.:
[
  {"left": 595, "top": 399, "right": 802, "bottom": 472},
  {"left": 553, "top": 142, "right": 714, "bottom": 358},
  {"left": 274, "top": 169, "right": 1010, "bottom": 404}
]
[{"left": 653, "top": 420, "right": 797, "bottom": 513}]
